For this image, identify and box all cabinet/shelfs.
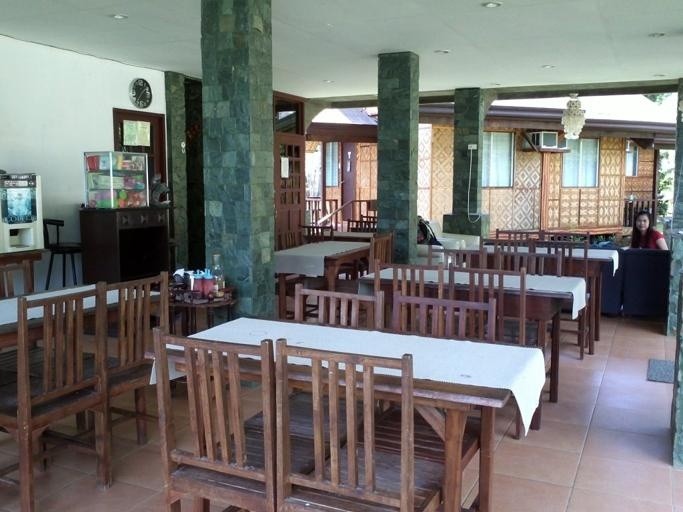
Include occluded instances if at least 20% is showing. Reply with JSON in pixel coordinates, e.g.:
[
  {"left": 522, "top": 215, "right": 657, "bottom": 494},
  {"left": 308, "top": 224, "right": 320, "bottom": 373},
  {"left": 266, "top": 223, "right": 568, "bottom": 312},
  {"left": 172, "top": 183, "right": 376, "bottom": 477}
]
[{"left": 83, "top": 150, "right": 149, "bottom": 209}]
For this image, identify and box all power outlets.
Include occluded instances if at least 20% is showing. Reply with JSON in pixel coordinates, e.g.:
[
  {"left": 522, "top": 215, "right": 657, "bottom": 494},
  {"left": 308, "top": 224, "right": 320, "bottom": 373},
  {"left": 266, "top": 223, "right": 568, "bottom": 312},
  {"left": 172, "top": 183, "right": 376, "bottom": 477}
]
[{"left": 468, "top": 144, "right": 477, "bottom": 151}]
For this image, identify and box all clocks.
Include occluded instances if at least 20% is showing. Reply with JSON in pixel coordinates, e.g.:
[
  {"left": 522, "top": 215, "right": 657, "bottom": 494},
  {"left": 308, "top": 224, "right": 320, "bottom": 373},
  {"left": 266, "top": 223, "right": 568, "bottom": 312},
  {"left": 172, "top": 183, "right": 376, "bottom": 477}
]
[{"left": 129, "top": 78, "right": 152, "bottom": 108}]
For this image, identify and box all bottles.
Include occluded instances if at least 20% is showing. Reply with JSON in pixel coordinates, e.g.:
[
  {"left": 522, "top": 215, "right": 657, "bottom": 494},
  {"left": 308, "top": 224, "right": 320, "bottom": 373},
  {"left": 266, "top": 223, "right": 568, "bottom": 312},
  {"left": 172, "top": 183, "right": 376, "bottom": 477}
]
[
  {"left": 193, "top": 268, "right": 213, "bottom": 297},
  {"left": 210, "top": 254, "right": 225, "bottom": 297},
  {"left": 456, "top": 239, "right": 466, "bottom": 251}
]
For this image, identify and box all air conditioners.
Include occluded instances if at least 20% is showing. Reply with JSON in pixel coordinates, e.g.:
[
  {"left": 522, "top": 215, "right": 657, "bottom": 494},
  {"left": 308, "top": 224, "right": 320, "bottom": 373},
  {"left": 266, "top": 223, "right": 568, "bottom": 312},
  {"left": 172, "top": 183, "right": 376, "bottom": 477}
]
[{"left": 528, "top": 131, "right": 560, "bottom": 150}]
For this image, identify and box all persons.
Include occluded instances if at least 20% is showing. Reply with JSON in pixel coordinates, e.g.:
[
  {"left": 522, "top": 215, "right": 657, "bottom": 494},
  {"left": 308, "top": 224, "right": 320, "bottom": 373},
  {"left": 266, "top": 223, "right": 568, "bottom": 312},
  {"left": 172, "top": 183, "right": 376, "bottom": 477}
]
[
  {"left": 631, "top": 211, "right": 669, "bottom": 250},
  {"left": 417, "top": 222, "right": 443, "bottom": 246}
]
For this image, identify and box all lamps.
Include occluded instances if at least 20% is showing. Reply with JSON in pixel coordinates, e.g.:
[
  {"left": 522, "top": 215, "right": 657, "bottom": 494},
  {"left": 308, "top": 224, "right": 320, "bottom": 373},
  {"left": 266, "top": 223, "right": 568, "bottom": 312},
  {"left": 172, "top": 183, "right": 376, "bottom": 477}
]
[{"left": 560, "top": 94, "right": 586, "bottom": 141}]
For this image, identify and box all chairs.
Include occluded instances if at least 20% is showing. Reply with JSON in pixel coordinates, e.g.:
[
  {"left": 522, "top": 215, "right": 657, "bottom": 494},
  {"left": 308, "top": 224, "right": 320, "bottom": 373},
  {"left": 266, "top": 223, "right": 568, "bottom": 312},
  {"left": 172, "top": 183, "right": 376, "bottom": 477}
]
[{"left": 622, "top": 249, "right": 671, "bottom": 319}]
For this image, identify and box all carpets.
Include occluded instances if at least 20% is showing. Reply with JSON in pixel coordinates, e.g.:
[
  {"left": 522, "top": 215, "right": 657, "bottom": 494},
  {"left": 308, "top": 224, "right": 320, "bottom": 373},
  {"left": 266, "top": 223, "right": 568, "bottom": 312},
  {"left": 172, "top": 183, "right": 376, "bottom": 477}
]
[{"left": 647, "top": 358, "right": 675, "bottom": 383}]
